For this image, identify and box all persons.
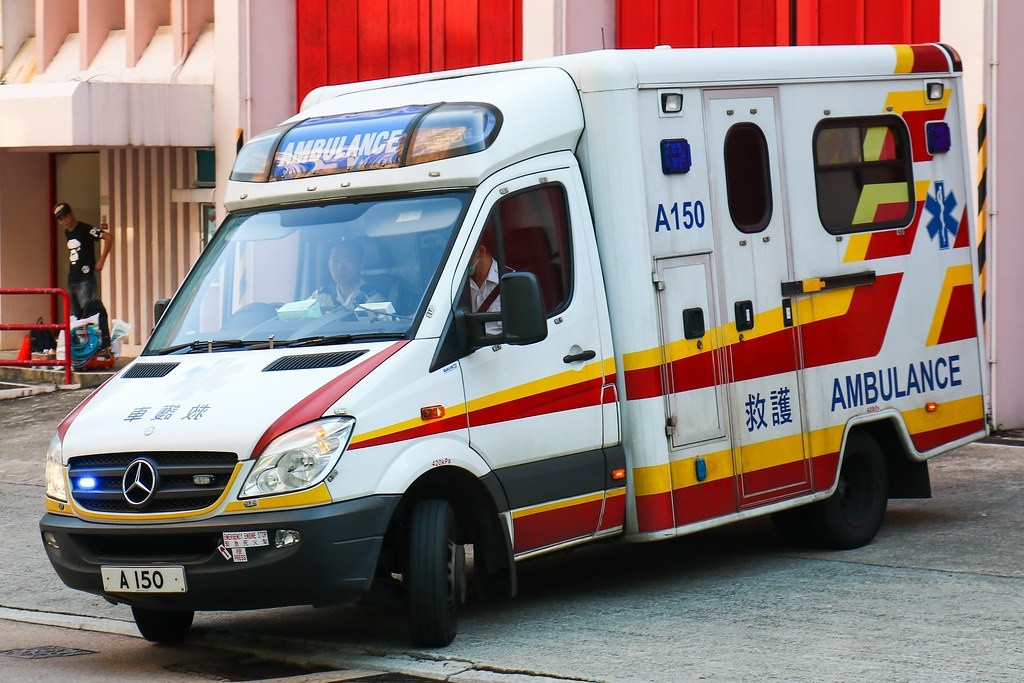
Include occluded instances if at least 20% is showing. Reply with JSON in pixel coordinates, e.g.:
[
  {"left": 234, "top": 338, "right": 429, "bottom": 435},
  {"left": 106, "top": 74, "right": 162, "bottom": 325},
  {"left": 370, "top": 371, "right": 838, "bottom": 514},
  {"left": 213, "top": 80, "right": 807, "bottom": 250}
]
[
  {"left": 398, "top": 216, "right": 516, "bottom": 335},
  {"left": 54, "top": 202, "right": 113, "bottom": 320},
  {"left": 307, "top": 242, "right": 385, "bottom": 309}
]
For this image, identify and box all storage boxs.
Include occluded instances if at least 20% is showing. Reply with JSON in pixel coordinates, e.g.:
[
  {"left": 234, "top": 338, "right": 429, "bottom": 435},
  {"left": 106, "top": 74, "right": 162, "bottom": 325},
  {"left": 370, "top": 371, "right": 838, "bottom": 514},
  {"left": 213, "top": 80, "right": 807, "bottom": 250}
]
[{"left": 30, "top": 352, "right": 56, "bottom": 360}]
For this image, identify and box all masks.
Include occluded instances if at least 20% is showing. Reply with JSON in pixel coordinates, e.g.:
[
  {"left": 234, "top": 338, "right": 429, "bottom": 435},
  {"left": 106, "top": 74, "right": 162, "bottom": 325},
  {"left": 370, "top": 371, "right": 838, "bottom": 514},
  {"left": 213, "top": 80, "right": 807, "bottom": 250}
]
[{"left": 469, "top": 259, "right": 478, "bottom": 276}]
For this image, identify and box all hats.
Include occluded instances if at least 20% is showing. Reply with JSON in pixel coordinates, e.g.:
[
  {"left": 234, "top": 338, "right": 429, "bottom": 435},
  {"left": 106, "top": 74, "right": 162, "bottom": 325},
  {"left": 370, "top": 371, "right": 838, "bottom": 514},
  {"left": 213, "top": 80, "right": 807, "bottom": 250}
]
[{"left": 52, "top": 202, "right": 71, "bottom": 219}]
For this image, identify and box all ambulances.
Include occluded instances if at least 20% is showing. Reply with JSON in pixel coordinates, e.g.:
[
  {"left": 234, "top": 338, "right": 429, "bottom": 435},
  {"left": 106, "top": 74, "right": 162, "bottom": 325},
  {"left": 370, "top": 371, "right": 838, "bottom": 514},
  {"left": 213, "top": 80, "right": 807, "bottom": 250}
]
[{"left": 39, "top": 39, "right": 993, "bottom": 646}]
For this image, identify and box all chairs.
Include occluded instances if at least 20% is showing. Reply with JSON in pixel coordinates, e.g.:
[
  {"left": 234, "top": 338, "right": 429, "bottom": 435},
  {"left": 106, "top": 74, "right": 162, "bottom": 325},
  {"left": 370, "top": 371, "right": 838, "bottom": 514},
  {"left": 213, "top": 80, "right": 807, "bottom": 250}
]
[{"left": 499, "top": 224, "right": 560, "bottom": 311}]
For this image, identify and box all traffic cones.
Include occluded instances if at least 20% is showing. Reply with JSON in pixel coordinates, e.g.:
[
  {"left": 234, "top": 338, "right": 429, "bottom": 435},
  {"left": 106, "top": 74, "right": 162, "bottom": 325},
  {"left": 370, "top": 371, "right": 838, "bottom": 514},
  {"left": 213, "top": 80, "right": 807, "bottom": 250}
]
[{"left": 15, "top": 335, "right": 32, "bottom": 368}]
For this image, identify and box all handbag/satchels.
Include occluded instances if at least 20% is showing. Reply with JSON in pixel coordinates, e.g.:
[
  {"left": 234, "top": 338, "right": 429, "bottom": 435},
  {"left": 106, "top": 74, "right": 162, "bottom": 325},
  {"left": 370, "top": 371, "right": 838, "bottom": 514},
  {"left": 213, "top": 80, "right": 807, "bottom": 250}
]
[{"left": 31, "top": 317, "right": 56, "bottom": 351}]
